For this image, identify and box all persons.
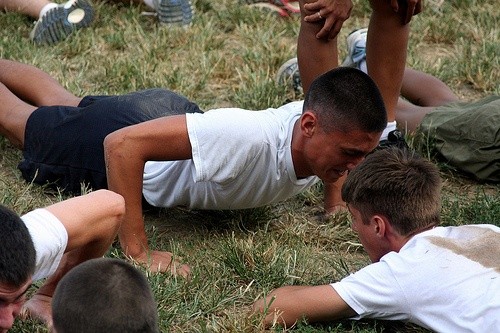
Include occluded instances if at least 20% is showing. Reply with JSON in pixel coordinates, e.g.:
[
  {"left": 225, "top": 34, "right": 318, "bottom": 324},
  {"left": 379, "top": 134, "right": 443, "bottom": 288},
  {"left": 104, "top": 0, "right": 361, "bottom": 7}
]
[
  {"left": 253, "top": 146, "right": 500, "bottom": 333},
  {"left": 0, "top": 59, "right": 388, "bottom": 284},
  {"left": 51, "top": 258, "right": 159, "bottom": 333},
  {"left": 296, "top": 0, "right": 422, "bottom": 158},
  {"left": 274, "top": 26, "right": 500, "bottom": 185},
  {"left": 0, "top": 0, "right": 195, "bottom": 48},
  {"left": 0, "top": 189, "right": 126, "bottom": 333}
]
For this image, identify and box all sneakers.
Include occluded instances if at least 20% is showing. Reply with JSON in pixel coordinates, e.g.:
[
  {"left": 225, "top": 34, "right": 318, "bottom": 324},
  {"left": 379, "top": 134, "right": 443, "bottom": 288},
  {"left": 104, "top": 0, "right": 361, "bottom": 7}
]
[
  {"left": 340, "top": 27, "right": 368, "bottom": 69},
  {"left": 275, "top": 57, "right": 303, "bottom": 104}
]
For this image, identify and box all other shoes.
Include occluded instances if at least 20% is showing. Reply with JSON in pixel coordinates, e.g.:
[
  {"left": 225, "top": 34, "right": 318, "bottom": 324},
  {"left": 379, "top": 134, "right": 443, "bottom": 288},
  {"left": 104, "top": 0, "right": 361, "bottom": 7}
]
[
  {"left": 158, "top": 0, "right": 196, "bottom": 29},
  {"left": 364, "top": 129, "right": 411, "bottom": 159},
  {"left": 30, "top": 0, "right": 95, "bottom": 47}
]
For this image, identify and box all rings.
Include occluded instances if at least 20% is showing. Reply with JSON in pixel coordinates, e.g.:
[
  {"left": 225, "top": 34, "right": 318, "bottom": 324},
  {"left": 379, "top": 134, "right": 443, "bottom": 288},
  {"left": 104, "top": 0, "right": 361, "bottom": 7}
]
[{"left": 317, "top": 11, "right": 322, "bottom": 19}]
